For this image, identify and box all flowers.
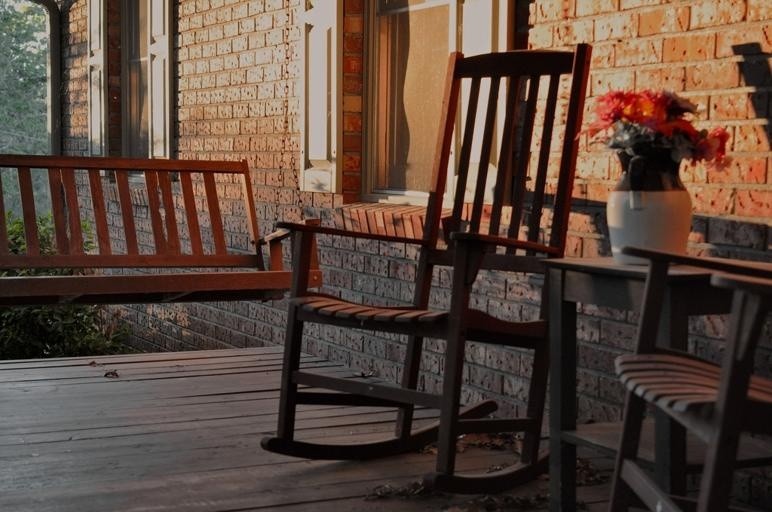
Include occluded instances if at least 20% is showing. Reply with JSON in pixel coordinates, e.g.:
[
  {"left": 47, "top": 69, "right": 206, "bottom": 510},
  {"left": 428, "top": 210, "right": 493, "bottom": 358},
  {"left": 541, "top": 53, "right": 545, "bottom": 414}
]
[{"left": 574, "top": 86, "right": 734, "bottom": 171}]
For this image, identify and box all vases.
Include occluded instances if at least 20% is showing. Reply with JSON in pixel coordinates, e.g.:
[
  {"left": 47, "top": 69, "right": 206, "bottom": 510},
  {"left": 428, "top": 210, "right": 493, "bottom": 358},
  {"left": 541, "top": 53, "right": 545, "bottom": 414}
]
[{"left": 607, "top": 147, "right": 693, "bottom": 267}]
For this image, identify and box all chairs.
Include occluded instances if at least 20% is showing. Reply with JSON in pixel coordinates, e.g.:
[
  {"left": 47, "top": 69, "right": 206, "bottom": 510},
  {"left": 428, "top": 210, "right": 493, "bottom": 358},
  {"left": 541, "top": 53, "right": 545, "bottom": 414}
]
[
  {"left": 608, "top": 246, "right": 772, "bottom": 511},
  {"left": 259, "top": 42, "right": 593, "bottom": 494}
]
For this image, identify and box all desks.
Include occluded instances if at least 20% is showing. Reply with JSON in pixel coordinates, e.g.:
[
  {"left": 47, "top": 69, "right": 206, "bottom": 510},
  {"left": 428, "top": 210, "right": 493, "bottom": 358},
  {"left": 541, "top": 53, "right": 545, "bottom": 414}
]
[{"left": 540, "top": 258, "right": 730, "bottom": 512}]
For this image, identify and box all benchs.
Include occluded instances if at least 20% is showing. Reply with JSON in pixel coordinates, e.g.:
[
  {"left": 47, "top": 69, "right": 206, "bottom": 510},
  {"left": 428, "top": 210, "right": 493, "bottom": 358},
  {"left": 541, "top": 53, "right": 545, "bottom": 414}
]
[{"left": 0, "top": 153, "right": 324, "bottom": 299}]
